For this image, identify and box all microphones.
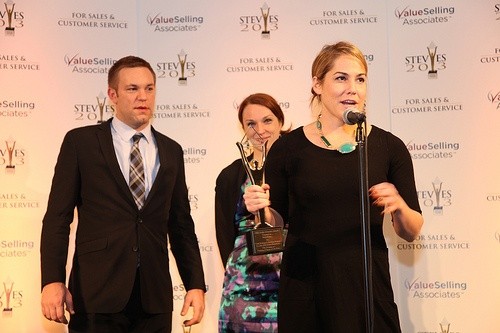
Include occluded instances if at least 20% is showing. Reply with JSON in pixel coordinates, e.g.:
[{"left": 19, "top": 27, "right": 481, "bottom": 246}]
[{"left": 343, "top": 107, "right": 366, "bottom": 125}]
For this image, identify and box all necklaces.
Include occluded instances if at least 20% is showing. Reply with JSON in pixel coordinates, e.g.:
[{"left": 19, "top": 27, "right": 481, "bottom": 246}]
[{"left": 316, "top": 113, "right": 357, "bottom": 153}]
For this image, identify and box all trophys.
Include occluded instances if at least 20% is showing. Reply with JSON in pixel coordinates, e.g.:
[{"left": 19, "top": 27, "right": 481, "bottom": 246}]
[{"left": 235, "top": 123, "right": 285, "bottom": 257}]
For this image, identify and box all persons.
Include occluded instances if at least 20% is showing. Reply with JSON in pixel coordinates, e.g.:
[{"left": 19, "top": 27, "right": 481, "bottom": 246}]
[
  {"left": 214, "top": 94, "right": 294, "bottom": 333},
  {"left": 39, "top": 56, "right": 208, "bottom": 333},
  {"left": 242, "top": 40, "right": 424, "bottom": 333}
]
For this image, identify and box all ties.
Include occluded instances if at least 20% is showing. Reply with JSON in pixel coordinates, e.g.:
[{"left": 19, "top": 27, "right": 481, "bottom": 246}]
[{"left": 130, "top": 134, "right": 146, "bottom": 211}]
